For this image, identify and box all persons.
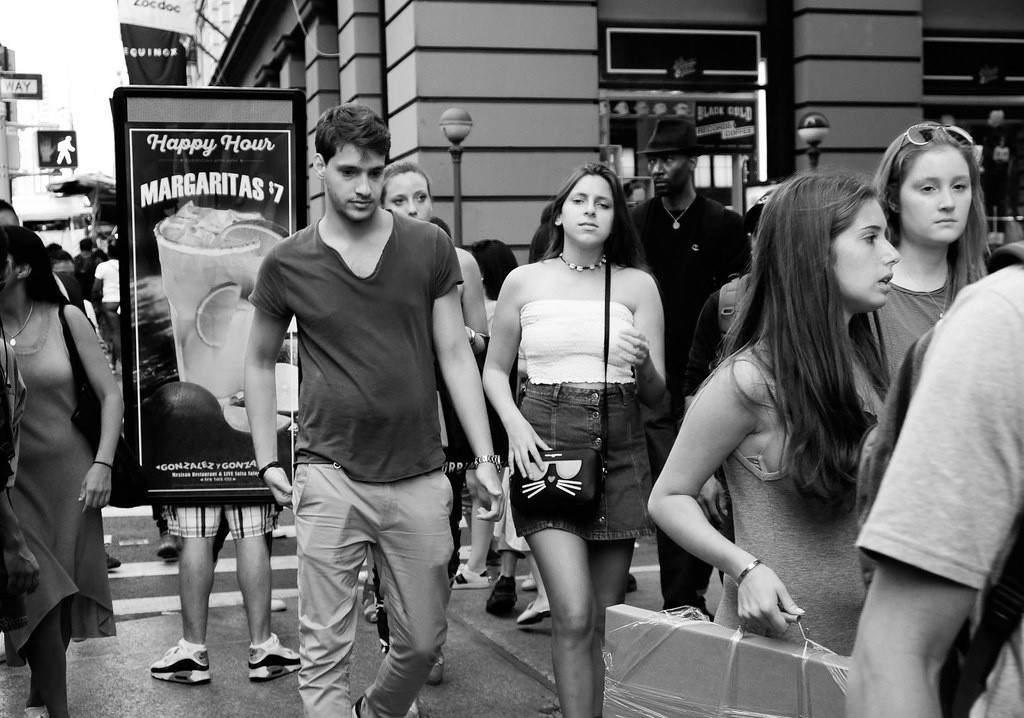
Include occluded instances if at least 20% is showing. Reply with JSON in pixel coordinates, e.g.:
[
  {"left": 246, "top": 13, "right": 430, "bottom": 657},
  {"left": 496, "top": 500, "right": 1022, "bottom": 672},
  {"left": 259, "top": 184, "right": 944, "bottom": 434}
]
[
  {"left": 148, "top": 504, "right": 302, "bottom": 683},
  {"left": 451, "top": 160, "right": 667, "bottom": 718},
  {"left": 0, "top": 199, "right": 182, "bottom": 718},
  {"left": 619, "top": 112, "right": 1024, "bottom": 717},
  {"left": 243, "top": 96, "right": 550, "bottom": 717}
]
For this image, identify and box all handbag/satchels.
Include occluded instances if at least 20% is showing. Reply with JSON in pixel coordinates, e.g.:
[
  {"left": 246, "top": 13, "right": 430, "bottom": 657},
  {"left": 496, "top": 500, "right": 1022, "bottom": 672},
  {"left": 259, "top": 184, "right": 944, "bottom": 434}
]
[
  {"left": 513, "top": 448, "right": 602, "bottom": 516},
  {"left": 57, "top": 304, "right": 148, "bottom": 508}
]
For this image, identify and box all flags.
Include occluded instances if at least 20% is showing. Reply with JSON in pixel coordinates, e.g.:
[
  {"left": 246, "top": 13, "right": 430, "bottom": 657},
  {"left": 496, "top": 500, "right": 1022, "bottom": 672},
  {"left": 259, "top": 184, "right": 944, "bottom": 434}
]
[{"left": 117, "top": 0, "right": 197, "bottom": 87}]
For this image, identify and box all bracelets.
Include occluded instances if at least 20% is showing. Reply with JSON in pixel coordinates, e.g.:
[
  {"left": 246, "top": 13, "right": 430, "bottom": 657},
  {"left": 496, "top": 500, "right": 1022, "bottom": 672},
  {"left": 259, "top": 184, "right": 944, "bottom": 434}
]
[
  {"left": 91, "top": 460, "right": 114, "bottom": 469},
  {"left": 735, "top": 560, "right": 762, "bottom": 586},
  {"left": 256, "top": 461, "right": 284, "bottom": 479}
]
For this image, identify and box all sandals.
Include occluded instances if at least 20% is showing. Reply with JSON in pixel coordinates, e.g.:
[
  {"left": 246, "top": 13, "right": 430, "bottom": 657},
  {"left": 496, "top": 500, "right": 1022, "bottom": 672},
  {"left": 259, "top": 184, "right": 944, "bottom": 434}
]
[{"left": 362, "top": 578, "right": 378, "bottom": 622}]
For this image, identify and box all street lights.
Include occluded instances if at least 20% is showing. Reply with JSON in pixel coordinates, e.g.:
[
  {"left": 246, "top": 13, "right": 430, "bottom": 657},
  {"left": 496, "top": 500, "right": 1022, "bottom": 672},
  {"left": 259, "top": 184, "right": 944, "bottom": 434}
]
[
  {"left": 797, "top": 111, "right": 830, "bottom": 171},
  {"left": 439, "top": 107, "right": 474, "bottom": 249}
]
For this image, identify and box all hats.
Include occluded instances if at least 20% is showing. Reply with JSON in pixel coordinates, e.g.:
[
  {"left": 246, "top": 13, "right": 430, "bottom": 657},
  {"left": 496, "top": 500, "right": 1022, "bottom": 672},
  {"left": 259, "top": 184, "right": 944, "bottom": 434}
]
[{"left": 636, "top": 113, "right": 714, "bottom": 154}]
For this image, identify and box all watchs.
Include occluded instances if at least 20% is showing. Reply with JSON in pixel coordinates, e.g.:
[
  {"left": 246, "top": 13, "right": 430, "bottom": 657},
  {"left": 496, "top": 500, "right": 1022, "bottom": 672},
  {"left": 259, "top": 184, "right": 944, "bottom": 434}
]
[
  {"left": 470, "top": 329, "right": 476, "bottom": 345},
  {"left": 472, "top": 453, "right": 503, "bottom": 472}
]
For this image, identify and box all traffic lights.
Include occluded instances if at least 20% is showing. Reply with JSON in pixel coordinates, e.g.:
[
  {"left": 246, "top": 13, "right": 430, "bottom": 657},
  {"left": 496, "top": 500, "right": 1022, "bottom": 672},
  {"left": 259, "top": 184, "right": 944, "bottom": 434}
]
[{"left": 37, "top": 129, "right": 78, "bottom": 169}]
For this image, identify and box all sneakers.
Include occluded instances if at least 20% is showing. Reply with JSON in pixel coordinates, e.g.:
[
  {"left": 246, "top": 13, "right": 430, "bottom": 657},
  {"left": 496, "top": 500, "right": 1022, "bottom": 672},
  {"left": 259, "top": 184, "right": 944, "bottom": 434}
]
[
  {"left": 247, "top": 630, "right": 303, "bottom": 679},
  {"left": 151, "top": 642, "right": 211, "bottom": 683},
  {"left": 452, "top": 564, "right": 490, "bottom": 589}
]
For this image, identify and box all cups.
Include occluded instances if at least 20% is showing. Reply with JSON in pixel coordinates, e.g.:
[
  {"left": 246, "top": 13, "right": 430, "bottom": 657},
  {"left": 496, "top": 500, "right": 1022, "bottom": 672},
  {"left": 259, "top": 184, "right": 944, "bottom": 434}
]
[{"left": 154, "top": 213, "right": 267, "bottom": 412}]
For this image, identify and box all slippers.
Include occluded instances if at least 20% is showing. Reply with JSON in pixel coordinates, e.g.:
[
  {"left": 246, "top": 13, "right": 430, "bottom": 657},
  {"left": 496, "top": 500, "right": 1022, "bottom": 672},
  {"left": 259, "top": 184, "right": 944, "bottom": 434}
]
[{"left": 517, "top": 600, "right": 552, "bottom": 625}]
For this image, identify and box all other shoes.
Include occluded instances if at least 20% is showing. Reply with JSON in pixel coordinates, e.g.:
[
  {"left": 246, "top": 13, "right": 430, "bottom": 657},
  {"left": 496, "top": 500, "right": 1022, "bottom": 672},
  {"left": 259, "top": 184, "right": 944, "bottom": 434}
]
[
  {"left": 23, "top": 705, "right": 50, "bottom": 718},
  {"left": 271, "top": 598, "right": 286, "bottom": 612},
  {"left": 486, "top": 576, "right": 516, "bottom": 615},
  {"left": 625, "top": 574, "right": 637, "bottom": 592},
  {"left": 350, "top": 696, "right": 364, "bottom": 718},
  {"left": 521, "top": 571, "right": 535, "bottom": 590},
  {"left": 0, "top": 631, "right": 6, "bottom": 662},
  {"left": 156, "top": 533, "right": 180, "bottom": 561},
  {"left": 106, "top": 550, "right": 121, "bottom": 569},
  {"left": 426, "top": 656, "right": 445, "bottom": 681},
  {"left": 539, "top": 700, "right": 565, "bottom": 718}
]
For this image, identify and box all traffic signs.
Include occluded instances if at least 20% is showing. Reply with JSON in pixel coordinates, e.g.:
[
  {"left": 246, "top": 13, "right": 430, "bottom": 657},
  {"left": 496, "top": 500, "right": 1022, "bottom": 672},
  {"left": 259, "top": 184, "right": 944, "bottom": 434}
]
[{"left": 0, "top": 72, "right": 44, "bottom": 102}]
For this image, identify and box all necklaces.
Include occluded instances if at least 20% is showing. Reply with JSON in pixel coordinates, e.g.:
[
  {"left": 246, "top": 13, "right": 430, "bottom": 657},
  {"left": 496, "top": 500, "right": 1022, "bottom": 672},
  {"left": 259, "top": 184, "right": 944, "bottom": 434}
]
[
  {"left": 905, "top": 266, "right": 950, "bottom": 318},
  {"left": 558, "top": 251, "right": 608, "bottom": 272},
  {"left": 663, "top": 205, "right": 689, "bottom": 229}
]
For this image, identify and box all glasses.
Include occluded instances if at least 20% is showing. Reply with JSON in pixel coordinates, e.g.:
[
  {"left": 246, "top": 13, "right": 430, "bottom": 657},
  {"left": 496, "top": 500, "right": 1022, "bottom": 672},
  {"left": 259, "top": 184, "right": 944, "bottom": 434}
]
[{"left": 886, "top": 124, "right": 973, "bottom": 185}]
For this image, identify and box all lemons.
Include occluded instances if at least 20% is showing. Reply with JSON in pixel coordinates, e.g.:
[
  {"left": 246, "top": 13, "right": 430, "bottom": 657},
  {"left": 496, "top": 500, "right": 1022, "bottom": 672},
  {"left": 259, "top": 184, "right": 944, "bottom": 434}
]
[
  {"left": 195, "top": 281, "right": 242, "bottom": 348},
  {"left": 145, "top": 382, "right": 221, "bottom": 439},
  {"left": 223, "top": 406, "right": 292, "bottom": 435},
  {"left": 220, "top": 219, "right": 290, "bottom": 254}
]
